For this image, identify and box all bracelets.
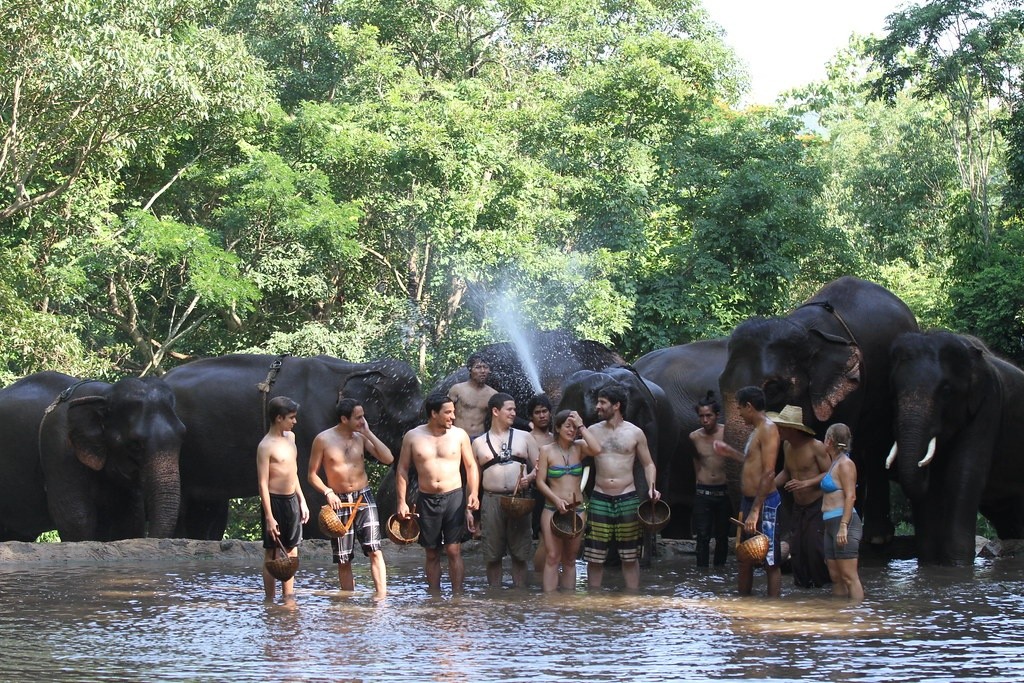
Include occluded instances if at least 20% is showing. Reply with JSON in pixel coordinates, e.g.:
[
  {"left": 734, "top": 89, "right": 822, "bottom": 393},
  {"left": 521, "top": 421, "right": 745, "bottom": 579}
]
[
  {"left": 840, "top": 522, "right": 848, "bottom": 524},
  {"left": 578, "top": 425, "right": 584, "bottom": 429},
  {"left": 324, "top": 489, "right": 332, "bottom": 495}
]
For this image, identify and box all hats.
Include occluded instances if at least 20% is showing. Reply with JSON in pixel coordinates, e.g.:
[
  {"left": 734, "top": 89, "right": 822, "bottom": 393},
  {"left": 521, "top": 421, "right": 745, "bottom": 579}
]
[{"left": 764, "top": 404, "right": 816, "bottom": 436}]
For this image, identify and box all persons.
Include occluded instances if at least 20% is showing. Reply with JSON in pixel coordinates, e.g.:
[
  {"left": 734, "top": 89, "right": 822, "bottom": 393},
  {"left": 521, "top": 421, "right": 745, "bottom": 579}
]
[
  {"left": 467, "top": 393, "right": 539, "bottom": 588},
  {"left": 820, "top": 423, "right": 865, "bottom": 598},
  {"left": 766, "top": 406, "right": 826, "bottom": 588},
  {"left": 396, "top": 394, "right": 479, "bottom": 591},
  {"left": 528, "top": 395, "right": 554, "bottom": 538},
  {"left": 447, "top": 353, "right": 534, "bottom": 445},
  {"left": 714, "top": 386, "right": 782, "bottom": 597},
  {"left": 307, "top": 398, "right": 394, "bottom": 591},
  {"left": 534, "top": 386, "right": 661, "bottom": 589},
  {"left": 257, "top": 397, "right": 310, "bottom": 597},
  {"left": 534, "top": 410, "right": 602, "bottom": 590},
  {"left": 689, "top": 391, "right": 731, "bottom": 567}
]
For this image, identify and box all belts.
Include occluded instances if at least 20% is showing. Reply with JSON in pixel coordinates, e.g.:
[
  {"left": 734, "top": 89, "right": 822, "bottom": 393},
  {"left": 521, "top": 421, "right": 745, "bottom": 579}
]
[{"left": 695, "top": 489, "right": 729, "bottom": 496}]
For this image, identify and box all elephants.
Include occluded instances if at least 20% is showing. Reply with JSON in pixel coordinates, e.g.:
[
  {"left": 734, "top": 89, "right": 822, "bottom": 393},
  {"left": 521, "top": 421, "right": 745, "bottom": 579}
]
[{"left": 0, "top": 275, "right": 1024, "bottom": 569}]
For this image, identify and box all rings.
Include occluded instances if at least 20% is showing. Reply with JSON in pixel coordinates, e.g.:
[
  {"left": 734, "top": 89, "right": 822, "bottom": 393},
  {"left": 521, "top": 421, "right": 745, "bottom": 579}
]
[{"left": 750, "top": 528, "right": 752, "bottom": 530}]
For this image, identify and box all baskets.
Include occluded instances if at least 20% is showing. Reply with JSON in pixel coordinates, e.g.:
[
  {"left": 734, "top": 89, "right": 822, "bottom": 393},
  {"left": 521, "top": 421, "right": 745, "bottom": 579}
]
[
  {"left": 317, "top": 495, "right": 369, "bottom": 538},
  {"left": 385, "top": 504, "right": 421, "bottom": 545},
  {"left": 550, "top": 492, "right": 585, "bottom": 539},
  {"left": 637, "top": 482, "right": 671, "bottom": 534},
  {"left": 729, "top": 511, "right": 769, "bottom": 563},
  {"left": 501, "top": 464, "right": 537, "bottom": 519},
  {"left": 265, "top": 525, "right": 299, "bottom": 583}
]
